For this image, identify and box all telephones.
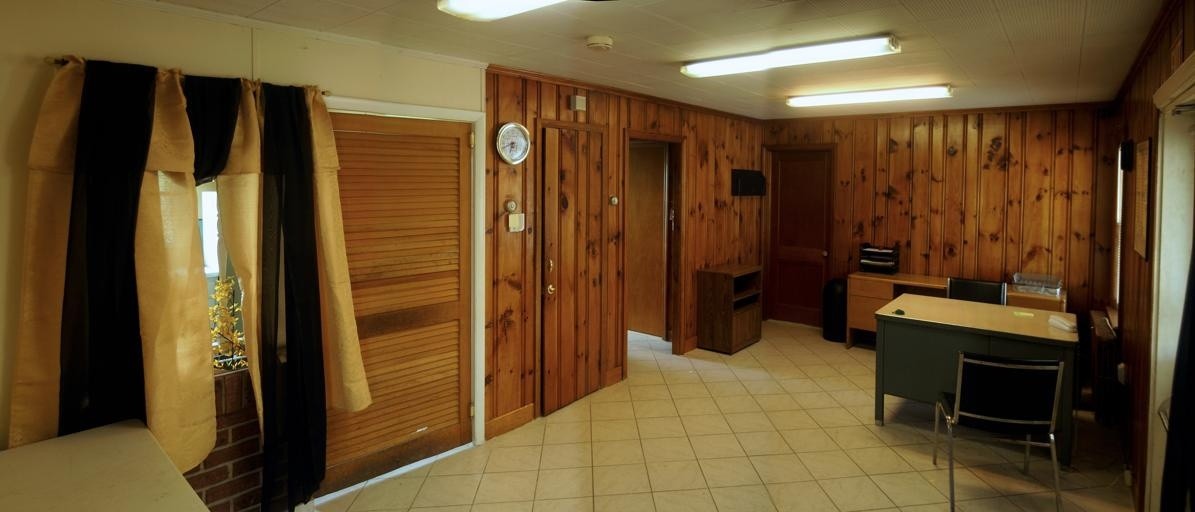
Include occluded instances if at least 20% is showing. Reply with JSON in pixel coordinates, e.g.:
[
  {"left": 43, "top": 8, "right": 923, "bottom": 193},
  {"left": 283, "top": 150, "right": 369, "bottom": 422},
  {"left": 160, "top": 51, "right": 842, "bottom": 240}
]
[{"left": 1048, "top": 315, "right": 1078, "bottom": 333}]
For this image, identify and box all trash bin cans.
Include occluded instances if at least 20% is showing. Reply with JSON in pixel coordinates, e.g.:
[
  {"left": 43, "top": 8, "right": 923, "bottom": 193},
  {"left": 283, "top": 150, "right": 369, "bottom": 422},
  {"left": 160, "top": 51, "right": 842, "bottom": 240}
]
[{"left": 822, "top": 278, "right": 848, "bottom": 343}]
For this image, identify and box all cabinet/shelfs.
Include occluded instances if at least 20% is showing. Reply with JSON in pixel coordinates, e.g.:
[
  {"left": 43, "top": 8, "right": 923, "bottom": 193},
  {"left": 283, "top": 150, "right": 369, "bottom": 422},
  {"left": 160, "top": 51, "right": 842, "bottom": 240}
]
[{"left": 696, "top": 262, "right": 764, "bottom": 356}]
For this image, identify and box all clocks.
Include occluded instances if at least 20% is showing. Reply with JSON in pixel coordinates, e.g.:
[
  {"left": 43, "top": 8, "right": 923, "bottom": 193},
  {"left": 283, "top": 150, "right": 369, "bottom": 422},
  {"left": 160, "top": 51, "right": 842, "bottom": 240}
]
[{"left": 495, "top": 119, "right": 532, "bottom": 167}]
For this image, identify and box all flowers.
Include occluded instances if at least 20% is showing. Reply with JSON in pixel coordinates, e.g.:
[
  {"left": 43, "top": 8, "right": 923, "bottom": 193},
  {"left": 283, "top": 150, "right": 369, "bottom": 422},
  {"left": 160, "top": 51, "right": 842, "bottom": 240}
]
[{"left": 207, "top": 273, "right": 248, "bottom": 371}]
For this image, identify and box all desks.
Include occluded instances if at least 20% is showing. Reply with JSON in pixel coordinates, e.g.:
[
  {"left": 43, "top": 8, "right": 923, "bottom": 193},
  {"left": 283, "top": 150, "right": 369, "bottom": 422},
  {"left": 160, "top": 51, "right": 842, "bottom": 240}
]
[
  {"left": 871, "top": 291, "right": 1081, "bottom": 472},
  {"left": 845, "top": 269, "right": 1070, "bottom": 350},
  {"left": 0, "top": 416, "right": 214, "bottom": 512}
]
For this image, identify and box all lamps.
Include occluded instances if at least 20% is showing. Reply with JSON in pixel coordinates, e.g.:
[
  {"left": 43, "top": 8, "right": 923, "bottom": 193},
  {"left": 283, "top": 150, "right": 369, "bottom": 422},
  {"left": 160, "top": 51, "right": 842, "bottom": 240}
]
[
  {"left": 434, "top": 2, "right": 596, "bottom": 22},
  {"left": 785, "top": 82, "right": 955, "bottom": 108},
  {"left": 679, "top": 30, "right": 903, "bottom": 81}
]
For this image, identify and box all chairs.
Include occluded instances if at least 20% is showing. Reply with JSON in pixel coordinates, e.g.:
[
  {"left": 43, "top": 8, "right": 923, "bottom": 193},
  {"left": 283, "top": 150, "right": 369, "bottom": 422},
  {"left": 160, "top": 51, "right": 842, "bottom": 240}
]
[
  {"left": 945, "top": 274, "right": 1008, "bottom": 306},
  {"left": 931, "top": 350, "right": 1066, "bottom": 512}
]
